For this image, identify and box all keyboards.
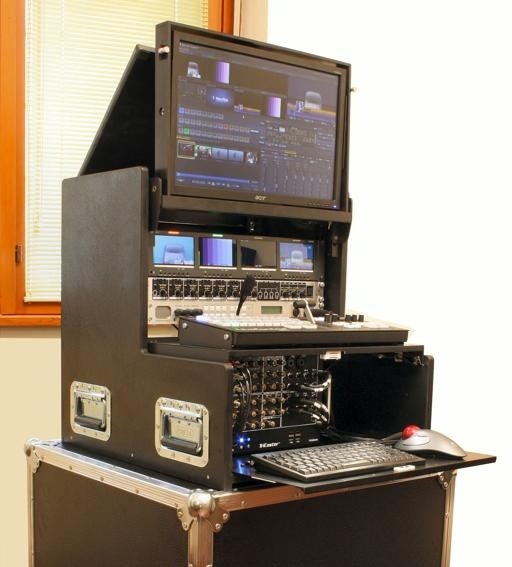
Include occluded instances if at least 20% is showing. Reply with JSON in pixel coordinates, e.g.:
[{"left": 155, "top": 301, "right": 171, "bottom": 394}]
[
  {"left": 251, "top": 441, "right": 426, "bottom": 483},
  {"left": 196, "top": 313, "right": 311, "bottom": 326}
]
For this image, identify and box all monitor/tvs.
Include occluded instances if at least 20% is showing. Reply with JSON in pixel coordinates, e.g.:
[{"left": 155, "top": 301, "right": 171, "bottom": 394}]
[{"left": 171, "top": 30, "right": 347, "bottom": 210}]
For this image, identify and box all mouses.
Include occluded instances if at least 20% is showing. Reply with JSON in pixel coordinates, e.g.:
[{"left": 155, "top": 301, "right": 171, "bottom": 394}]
[{"left": 393, "top": 425, "right": 467, "bottom": 460}]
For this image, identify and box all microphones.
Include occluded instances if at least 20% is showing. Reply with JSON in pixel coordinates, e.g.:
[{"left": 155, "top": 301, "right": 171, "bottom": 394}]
[{"left": 236, "top": 276, "right": 255, "bottom": 316}]
